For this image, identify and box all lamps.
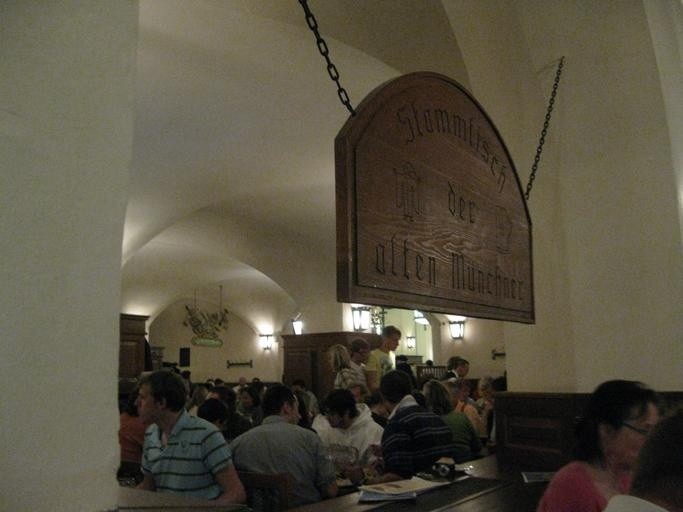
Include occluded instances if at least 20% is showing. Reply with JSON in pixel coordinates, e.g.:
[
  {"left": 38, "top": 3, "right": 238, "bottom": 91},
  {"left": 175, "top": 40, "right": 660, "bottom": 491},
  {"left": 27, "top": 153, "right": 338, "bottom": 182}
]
[{"left": 258, "top": 306, "right": 465, "bottom": 350}]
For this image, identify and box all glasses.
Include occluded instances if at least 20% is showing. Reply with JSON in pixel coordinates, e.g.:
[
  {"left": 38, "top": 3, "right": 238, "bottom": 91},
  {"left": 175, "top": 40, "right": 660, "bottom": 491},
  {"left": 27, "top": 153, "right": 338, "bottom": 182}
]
[
  {"left": 618, "top": 421, "right": 650, "bottom": 437},
  {"left": 357, "top": 352, "right": 369, "bottom": 356},
  {"left": 322, "top": 410, "right": 335, "bottom": 418}
]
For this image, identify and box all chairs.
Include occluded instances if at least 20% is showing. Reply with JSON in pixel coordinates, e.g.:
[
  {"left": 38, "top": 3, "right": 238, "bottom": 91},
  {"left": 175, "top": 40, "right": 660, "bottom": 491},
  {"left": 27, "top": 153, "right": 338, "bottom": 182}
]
[{"left": 238, "top": 471, "right": 293, "bottom": 512}]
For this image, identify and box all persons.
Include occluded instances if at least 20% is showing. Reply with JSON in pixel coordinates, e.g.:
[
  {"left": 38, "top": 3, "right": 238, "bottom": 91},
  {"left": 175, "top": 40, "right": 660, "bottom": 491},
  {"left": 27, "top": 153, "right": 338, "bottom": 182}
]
[
  {"left": 601, "top": 408, "right": 683, "bottom": 511},
  {"left": 118, "top": 325, "right": 498, "bottom": 503},
  {"left": 534, "top": 378, "right": 665, "bottom": 511}
]
